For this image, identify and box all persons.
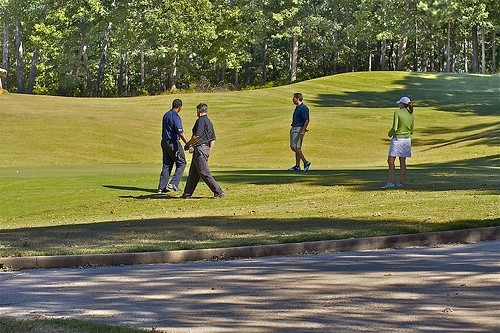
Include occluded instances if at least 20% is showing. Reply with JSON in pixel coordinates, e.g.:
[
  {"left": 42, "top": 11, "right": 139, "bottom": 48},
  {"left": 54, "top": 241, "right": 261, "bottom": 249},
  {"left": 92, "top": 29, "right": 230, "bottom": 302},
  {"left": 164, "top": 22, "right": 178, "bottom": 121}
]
[
  {"left": 158, "top": 98, "right": 188, "bottom": 194},
  {"left": 178, "top": 103, "right": 224, "bottom": 199},
  {"left": 381, "top": 97, "right": 414, "bottom": 189},
  {"left": 288, "top": 92, "right": 310, "bottom": 171}
]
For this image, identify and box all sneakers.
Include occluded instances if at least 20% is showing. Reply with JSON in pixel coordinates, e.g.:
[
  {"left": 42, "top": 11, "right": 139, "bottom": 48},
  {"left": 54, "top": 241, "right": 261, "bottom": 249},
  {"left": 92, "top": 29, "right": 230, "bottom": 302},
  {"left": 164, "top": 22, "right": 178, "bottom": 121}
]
[
  {"left": 288, "top": 166, "right": 300, "bottom": 171},
  {"left": 304, "top": 161, "right": 311, "bottom": 172}
]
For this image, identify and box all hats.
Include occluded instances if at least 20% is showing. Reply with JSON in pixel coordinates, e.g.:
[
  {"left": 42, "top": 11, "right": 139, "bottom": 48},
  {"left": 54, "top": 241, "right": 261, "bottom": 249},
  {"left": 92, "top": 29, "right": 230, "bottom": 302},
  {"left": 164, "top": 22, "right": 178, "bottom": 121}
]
[{"left": 396, "top": 96, "right": 410, "bottom": 104}]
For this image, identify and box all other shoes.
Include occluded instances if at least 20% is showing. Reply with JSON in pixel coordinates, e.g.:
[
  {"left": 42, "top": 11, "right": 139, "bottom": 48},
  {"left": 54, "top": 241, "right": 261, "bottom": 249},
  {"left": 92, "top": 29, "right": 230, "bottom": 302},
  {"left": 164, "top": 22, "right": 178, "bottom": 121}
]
[
  {"left": 213, "top": 191, "right": 224, "bottom": 199},
  {"left": 158, "top": 189, "right": 166, "bottom": 193},
  {"left": 168, "top": 184, "right": 177, "bottom": 191},
  {"left": 396, "top": 183, "right": 403, "bottom": 189},
  {"left": 182, "top": 194, "right": 191, "bottom": 199},
  {"left": 380, "top": 181, "right": 395, "bottom": 188}
]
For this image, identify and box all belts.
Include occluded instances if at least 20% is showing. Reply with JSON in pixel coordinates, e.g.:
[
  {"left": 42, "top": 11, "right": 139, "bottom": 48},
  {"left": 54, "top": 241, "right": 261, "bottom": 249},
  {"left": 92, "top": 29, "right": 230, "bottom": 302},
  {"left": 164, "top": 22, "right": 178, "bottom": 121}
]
[
  {"left": 291, "top": 124, "right": 303, "bottom": 127},
  {"left": 199, "top": 144, "right": 208, "bottom": 146}
]
